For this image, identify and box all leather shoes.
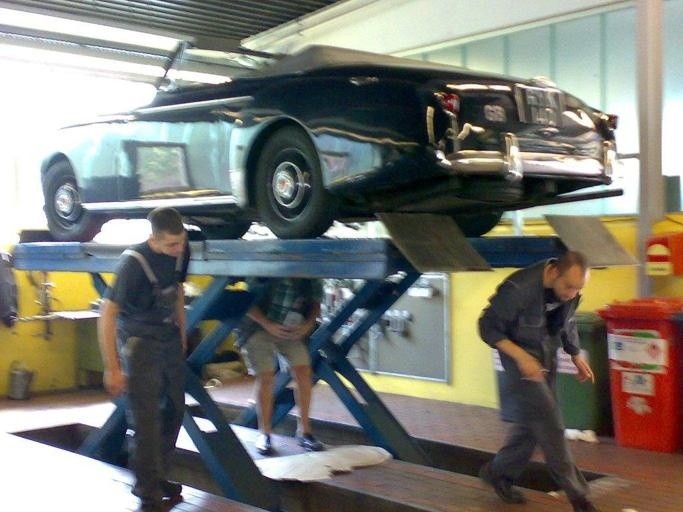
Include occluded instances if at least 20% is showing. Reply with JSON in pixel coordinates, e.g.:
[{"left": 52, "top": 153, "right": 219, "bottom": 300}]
[
  {"left": 571, "top": 500, "right": 596, "bottom": 511},
  {"left": 480, "top": 463, "right": 529, "bottom": 504}
]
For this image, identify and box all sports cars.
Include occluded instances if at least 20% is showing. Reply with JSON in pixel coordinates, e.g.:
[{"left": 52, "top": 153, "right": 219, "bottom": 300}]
[{"left": 41, "top": 41, "right": 618, "bottom": 239}]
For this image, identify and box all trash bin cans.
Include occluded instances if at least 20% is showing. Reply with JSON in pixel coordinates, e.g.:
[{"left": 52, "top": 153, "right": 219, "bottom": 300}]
[
  {"left": 497, "top": 299, "right": 683, "bottom": 454},
  {"left": 9, "top": 368, "right": 32, "bottom": 400}
]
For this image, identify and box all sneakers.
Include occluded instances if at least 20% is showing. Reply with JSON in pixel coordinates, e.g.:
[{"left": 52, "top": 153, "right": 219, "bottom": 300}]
[
  {"left": 131, "top": 479, "right": 182, "bottom": 497},
  {"left": 255, "top": 432, "right": 273, "bottom": 454},
  {"left": 297, "top": 433, "right": 324, "bottom": 450},
  {"left": 140, "top": 488, "right": 161, "bottom": 507}
]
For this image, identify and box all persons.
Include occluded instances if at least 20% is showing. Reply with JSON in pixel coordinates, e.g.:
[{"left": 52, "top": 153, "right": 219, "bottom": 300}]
[
  {"left": 229, "top": 276, "right": 326, "bottom": 458},
  {"left": 95, "top": 205, "right": 193, "bottom": 512},
  {"left": 477, "top": 248, "right": 601, "bottom": 511}
]
